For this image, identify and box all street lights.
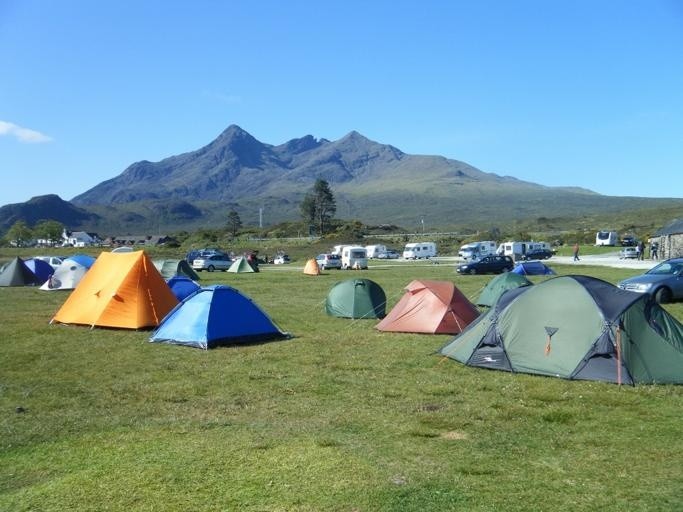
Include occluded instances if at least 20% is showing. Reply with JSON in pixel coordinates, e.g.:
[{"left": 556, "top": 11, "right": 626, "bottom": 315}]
[
  {"left": 420, "top": 219, "right": 424, "bottom": 234},
  {"left": 258, "top": 208, "right": 262, "bottom": 228}
]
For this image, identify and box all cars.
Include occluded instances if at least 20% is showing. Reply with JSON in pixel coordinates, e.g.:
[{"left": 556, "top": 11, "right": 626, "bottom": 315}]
[
  {"left": 457, "top": 254, "right": 514, "bottom": 276},
  {"left": 316, "top": 252, "right": 341, "bottom": 271},
  {"left": 617, "top": 234, "right": 682, "bottom": 304},
  {"left": 378, "top": 251, "right": 400, "bottom": 259},
  {"left": 414, "top": 231, "right": 418, "bottom": 234},
  {"left": 9, "top": 239, "right": 78, "bottom": 248},
  {"left": 186, "top": 249, "right": 289, "bottom": 273},
  {"left": 522, "top": 247, "right": 553, "bottom": 263}
]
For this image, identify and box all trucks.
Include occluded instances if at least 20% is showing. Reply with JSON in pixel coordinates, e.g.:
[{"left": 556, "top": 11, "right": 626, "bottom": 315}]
[
  {"left": 401, "top": 240, "right": 438, "bottom": 261},
  {"left": 454, "top": 238, "right": 500, "bottom": 259}
]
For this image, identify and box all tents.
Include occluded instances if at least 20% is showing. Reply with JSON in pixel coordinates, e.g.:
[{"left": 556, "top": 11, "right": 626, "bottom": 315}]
[
  {"left": 303, "top": 258, "right": 320, "bottom": 276},
  {"left": 147, "top": 284, "right": 291, "bottom": 352},
  {"left": 227, "top": 256, "right": 259, "bottom": 274},
  {"left": 372, "top": 279, "right": 481, "bottom": 335},
  {"left": 0, "top": 247, "right": 201, "bottom": 330},
  {"left": 435, "top": 275, "right": 683, "bottom": 385},
  {"left": 474, "top": 272, "right": 534, "bottom": 308},
  {"left": 648, "top": 218, "right": 683, "bottom": 260},
  {"left": 511, "top": 261, "right": 557, "bottom": 276},
  {"left": 325, "top": 278, "right": 386, "bottom": 319}
]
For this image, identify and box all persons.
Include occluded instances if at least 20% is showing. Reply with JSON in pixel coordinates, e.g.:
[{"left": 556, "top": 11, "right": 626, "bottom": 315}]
[
  {"left": 637, "top": 241, "right": 646, "bottom": 261},
  {"left": 573, "top": 243, "right": 581, "bottom": 261},
  {"left": 651, "top": 243, "right": 659, "bottom": 259}
]
